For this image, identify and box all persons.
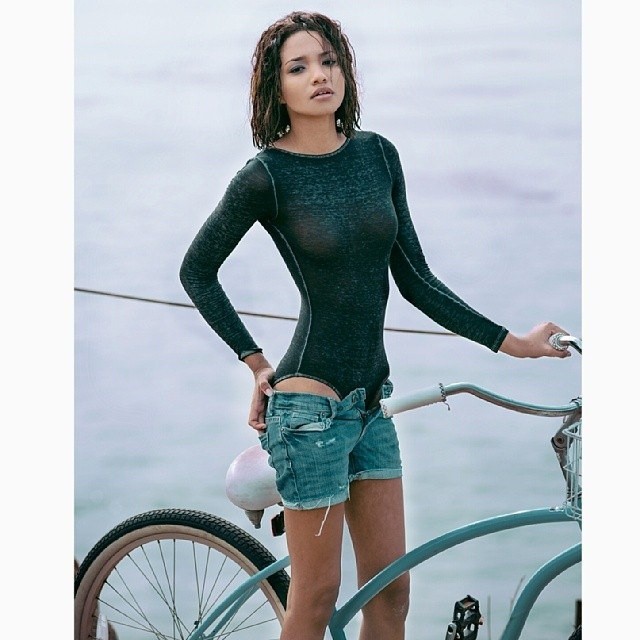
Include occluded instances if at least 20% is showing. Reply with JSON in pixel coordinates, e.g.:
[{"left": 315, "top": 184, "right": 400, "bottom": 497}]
[{"left": 178, "top": 11, "right": 573, "bottom": 639}]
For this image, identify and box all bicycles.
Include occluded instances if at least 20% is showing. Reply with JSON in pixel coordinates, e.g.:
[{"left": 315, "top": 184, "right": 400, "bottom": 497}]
[{"left": 74, "top": 332, "right": 582, "bottom": 639}]
